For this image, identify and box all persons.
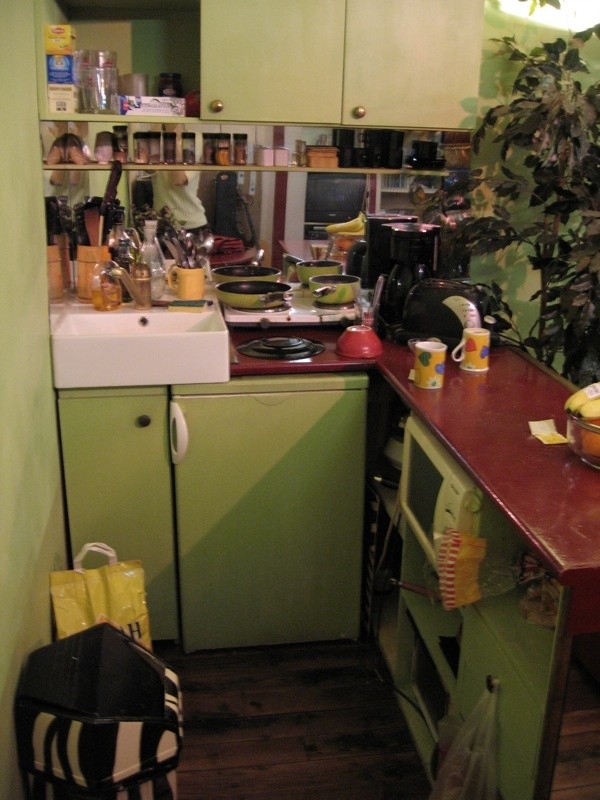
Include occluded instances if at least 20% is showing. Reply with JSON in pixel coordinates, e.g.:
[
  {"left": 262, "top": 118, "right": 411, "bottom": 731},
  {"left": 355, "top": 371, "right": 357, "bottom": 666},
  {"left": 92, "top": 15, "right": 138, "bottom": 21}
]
[{"left": 152, "top": 170, "right": 208, "bottom": 236}]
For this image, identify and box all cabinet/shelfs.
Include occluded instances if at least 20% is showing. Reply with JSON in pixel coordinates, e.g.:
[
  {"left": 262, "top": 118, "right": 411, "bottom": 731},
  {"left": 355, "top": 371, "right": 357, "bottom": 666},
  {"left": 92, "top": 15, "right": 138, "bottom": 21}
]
[
  {"left": 59, "top": 387, "right": 178, "bottom": 642},
  {"left": 372, "top": 368, "right": 600, "bottom": 800},
  {"left": 34, "top": 1, "right": 483, "bottom": 176},
  {"left": 170, "top": 372, "right": 368, "bottom": 651}
]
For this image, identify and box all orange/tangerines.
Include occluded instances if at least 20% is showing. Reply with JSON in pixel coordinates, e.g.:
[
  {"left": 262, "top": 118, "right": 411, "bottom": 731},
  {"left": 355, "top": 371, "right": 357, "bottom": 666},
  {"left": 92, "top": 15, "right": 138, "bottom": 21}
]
[{"left": 579, "top": 426, "right": 600, "bottom": 458}]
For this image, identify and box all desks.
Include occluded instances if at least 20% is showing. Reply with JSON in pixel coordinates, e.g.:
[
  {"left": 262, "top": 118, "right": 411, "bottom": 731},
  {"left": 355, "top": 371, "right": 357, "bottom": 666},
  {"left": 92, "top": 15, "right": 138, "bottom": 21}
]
[{"left": 164, "top": 235, "right": 260, "bottom": 267}]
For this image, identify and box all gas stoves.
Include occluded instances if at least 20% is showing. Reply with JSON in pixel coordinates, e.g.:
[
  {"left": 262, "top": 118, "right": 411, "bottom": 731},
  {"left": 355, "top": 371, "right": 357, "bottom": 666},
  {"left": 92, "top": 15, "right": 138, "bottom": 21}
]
[{"left": 221, "top": 280, "right": 376, "bottom": 328}]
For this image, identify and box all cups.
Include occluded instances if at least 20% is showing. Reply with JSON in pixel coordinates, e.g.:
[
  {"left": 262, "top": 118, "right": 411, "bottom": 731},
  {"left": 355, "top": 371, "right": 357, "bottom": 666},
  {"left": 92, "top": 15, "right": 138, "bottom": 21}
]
[
  {"left": 414, "top": 341, "right": 448, "bottom": 389},
  {"left": 167, "top": 262, "right": 206, "bottom": 300},
  {"left": 75, "top": 48, "right": 148, "bottom": 115},
  {"left": 332, "top": 128, "right": 406, "bottom": 169},
  {"left": 77, "top": 243, "right": 111, "bottom": 304},
  {"left": 451, "top": 327, "right": 491, "bottom": 372},
  {"left": 47, "top": 233, "right": 74, "bottom": 305}
]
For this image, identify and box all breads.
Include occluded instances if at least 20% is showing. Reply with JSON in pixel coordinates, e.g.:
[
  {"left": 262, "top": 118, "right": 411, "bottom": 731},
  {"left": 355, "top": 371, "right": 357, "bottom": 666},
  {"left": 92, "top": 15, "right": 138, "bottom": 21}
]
[{"left": 45, "top": 133, "right": 86, "bottom": 165}]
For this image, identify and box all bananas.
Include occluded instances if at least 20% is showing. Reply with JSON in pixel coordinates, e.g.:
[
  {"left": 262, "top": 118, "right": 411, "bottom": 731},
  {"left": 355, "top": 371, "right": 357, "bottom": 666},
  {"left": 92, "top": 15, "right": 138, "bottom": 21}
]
[
  {"left": 565, "top": 381, "right": 600, "bottom": 420},
  {"left": 324, "top": 214, "right": 366, "bottom": 233}
]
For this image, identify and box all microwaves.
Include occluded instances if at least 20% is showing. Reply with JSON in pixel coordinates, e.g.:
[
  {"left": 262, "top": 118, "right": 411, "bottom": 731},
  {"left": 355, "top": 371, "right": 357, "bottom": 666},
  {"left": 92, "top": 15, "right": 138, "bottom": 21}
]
[{"left": 396, "top": 414, "right": 477, "bottom": 577}]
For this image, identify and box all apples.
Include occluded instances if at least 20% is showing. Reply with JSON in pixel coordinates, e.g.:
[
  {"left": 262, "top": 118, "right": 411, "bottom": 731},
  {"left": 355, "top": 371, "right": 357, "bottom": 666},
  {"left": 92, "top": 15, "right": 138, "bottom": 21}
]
[{"left": 335, "top": 237, "right": 354, "bottom": 251}]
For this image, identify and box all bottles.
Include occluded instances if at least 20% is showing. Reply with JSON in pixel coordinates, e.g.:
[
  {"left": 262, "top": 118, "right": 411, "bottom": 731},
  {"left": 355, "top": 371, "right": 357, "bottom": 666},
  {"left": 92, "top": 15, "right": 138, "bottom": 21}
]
[
  {"left": 106, "top": 208, "right": 169, "bottom": 310},
  {"left": 255, "top": 145, "right": 290, "bottom": 167},
  {"left": 89, "top": 260, "right": 123, "bottom": 312},
  {"left": 94, "top": 125, "right": 248, "bottom": 166},
  {"left": 157, "top": 71, "right": 184, "bottom": 98}
]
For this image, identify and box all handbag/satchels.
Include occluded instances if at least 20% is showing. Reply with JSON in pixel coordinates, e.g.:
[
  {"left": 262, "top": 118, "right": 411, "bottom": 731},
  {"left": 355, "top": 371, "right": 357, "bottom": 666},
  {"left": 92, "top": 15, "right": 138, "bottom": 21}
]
[{"left": 49, "top": 542, "right": 152, "bottom": 654}]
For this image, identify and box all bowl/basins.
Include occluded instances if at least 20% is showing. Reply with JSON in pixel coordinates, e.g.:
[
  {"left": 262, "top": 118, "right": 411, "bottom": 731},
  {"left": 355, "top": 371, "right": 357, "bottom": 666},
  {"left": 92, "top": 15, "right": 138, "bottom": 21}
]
[
  {"left": 334, "top": 324, "right": 383, "bottom": 358},
  {"left": 565, "top": 408, "right": 600, "bottom": 469}
]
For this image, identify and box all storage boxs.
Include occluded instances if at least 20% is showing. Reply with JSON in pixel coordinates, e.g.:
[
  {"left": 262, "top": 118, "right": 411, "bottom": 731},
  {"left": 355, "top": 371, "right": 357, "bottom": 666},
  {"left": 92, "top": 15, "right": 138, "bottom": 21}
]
[
  {"left": 44, "top": 25, "right": 79, "bottom": 55},
  {"left": 48, "top": 84, "right": 81, "bottom": 112},
  {"left": 47, "top": 53, "right": 81, "bottom": 84},
  {"left": 110, "top": 92, "right": 186, "bottom": 117}
]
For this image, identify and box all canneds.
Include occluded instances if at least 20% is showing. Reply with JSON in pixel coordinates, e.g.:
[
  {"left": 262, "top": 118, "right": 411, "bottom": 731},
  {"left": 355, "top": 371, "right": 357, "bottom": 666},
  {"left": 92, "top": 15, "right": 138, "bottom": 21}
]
[{"left": 156, "top": 72, "right": 182, "bottom": 96}]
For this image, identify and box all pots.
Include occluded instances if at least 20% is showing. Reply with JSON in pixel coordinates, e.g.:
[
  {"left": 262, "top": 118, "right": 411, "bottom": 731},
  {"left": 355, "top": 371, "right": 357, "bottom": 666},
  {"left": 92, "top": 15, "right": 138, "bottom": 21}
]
[{"left": 210, "top": 253, "right": 376, "bottom": 312}]
